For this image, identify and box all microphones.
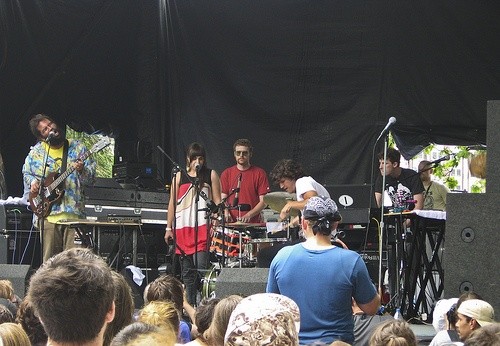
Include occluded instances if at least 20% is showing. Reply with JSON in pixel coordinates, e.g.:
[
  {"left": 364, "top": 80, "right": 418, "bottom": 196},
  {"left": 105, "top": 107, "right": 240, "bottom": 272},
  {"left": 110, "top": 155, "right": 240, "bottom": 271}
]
[
  {"left": 44, "top": 131, "right": 55, "bottom": 142},
  {"left": 378, "top": 116, "right": 397, "bottom": 141},
  {"left": 194, "top": 164, "right": 200, "bottom": 170},
  {"left": 430, "top": 156, "right": 450, "bottom": 164},
  {"left": 234, "top": 175, "right": 241, "bottom": 206},
  {"left": 232, "top": 203, "right": 251, "bottom": 211}
]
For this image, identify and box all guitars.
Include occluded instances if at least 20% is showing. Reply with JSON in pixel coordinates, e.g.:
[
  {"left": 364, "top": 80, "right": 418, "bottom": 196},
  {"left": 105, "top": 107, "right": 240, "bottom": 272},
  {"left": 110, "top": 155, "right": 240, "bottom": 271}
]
[{"left": 28, "top": 136, "right": 112, "bottom": 220}]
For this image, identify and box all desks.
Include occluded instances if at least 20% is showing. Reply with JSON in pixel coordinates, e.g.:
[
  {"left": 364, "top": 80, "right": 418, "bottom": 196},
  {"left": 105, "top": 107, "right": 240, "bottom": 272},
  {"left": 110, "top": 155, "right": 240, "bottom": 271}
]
[{"left": 383, "top": 212, "right": 446, "bottom": 323}]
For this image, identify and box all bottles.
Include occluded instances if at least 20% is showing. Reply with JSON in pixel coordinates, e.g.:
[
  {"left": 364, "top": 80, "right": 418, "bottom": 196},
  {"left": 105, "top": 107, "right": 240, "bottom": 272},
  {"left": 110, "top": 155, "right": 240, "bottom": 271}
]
[{"left": 394, "top": 309, "right": 403, "bottom": 320}]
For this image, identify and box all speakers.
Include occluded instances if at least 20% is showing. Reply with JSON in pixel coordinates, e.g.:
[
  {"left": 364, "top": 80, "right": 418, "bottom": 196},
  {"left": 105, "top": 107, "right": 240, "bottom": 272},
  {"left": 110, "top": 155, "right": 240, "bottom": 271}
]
[
  {"left": 0, "top": 263, "right": 32, "bottom": 299},
  {"left": 444, "top": 192, "right": 500, "bottom": 323},
  {"left": 0, "top": 204, "right": 42, "bottom": 271},
  {"left": 215, "top": 268, "right": 270, "bottom": 301}
]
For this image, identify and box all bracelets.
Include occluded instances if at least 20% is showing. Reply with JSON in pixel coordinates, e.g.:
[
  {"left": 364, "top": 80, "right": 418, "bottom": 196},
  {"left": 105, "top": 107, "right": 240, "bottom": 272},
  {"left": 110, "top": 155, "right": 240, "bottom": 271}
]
[{"left": 166, "top": 228, "right": 171, "bottom": 231}]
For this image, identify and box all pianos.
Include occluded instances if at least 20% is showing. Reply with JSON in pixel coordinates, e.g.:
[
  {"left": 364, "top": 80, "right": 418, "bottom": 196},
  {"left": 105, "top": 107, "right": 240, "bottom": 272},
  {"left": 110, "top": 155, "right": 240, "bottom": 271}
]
[
  {"left": 72, "top": 214, "right": 143, "bottom": 270},
  {"left": 381, "top": 209, "right": 446, "bottom": 323}
]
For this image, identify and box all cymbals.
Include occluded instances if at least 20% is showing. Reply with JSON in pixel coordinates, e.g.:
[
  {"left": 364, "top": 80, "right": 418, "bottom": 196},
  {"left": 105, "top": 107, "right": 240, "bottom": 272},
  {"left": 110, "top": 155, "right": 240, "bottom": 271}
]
[
  {"left": 262, "top": 192, "right": 299, "bottom": 216},
  {"left": 216, "top": 221, "right": 263, "bottom": 228}
]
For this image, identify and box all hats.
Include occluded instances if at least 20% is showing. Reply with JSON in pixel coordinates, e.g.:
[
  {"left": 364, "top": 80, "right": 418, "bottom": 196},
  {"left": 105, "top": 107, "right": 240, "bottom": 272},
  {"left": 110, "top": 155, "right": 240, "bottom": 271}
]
[
  {"left": 458, "top": 299, "right": 496, "bottom": 327},
  {"left": 224, "top": 292, "right": 301, "bottom": 346}
]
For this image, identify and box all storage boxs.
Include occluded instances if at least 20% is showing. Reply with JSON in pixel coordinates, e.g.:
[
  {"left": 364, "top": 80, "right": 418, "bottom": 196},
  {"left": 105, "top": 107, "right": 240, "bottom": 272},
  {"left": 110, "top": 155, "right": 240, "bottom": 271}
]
[{"left": 84, "top": 204, "right": 168, "bottom": 225}]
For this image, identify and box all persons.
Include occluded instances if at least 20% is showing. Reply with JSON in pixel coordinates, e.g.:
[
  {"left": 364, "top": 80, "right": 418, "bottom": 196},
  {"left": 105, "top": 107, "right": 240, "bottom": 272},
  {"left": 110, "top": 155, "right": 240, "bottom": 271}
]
[
  {"left": 220, "top": 139, "right": 272, "bottom": 268},
  {"left": 165, "top": 142, "right": 222, "bottom": 324},
  {"left": 21, "top": 113, "right": 96, "bottom": 265},
  {"left": 373, "top": 147, "right": 426, "bottom": 316},
  {"left": 0, "top": 247, "right": 301, "bottom": 346},
  {"left": 266, "top": 196, "right": 380, "bottom": 346},
  {"left": 418, "top": 160, "right": 450, "bottom": 211},
  {"left": 257, "top": 159, "right": 338, "bottom": 245},
  {"left": 368, "top": 292, "right": 500, "bottom": 346}
]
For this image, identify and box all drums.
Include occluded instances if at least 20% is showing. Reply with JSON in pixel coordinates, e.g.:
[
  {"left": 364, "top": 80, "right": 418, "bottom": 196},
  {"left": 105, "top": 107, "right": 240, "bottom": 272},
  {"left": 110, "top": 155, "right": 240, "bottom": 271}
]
[
  {"left": 243, "top": 237, "right": 286, "bottom": 265},
  {"left": 209, "top": 227, "right": 238, "bottom": 258},
  {"left": 225, "top": 232, "right": 251, "bottom": 260},
  {"left": 201, "top": 261, "right": 224, "bottom": 301}
]
